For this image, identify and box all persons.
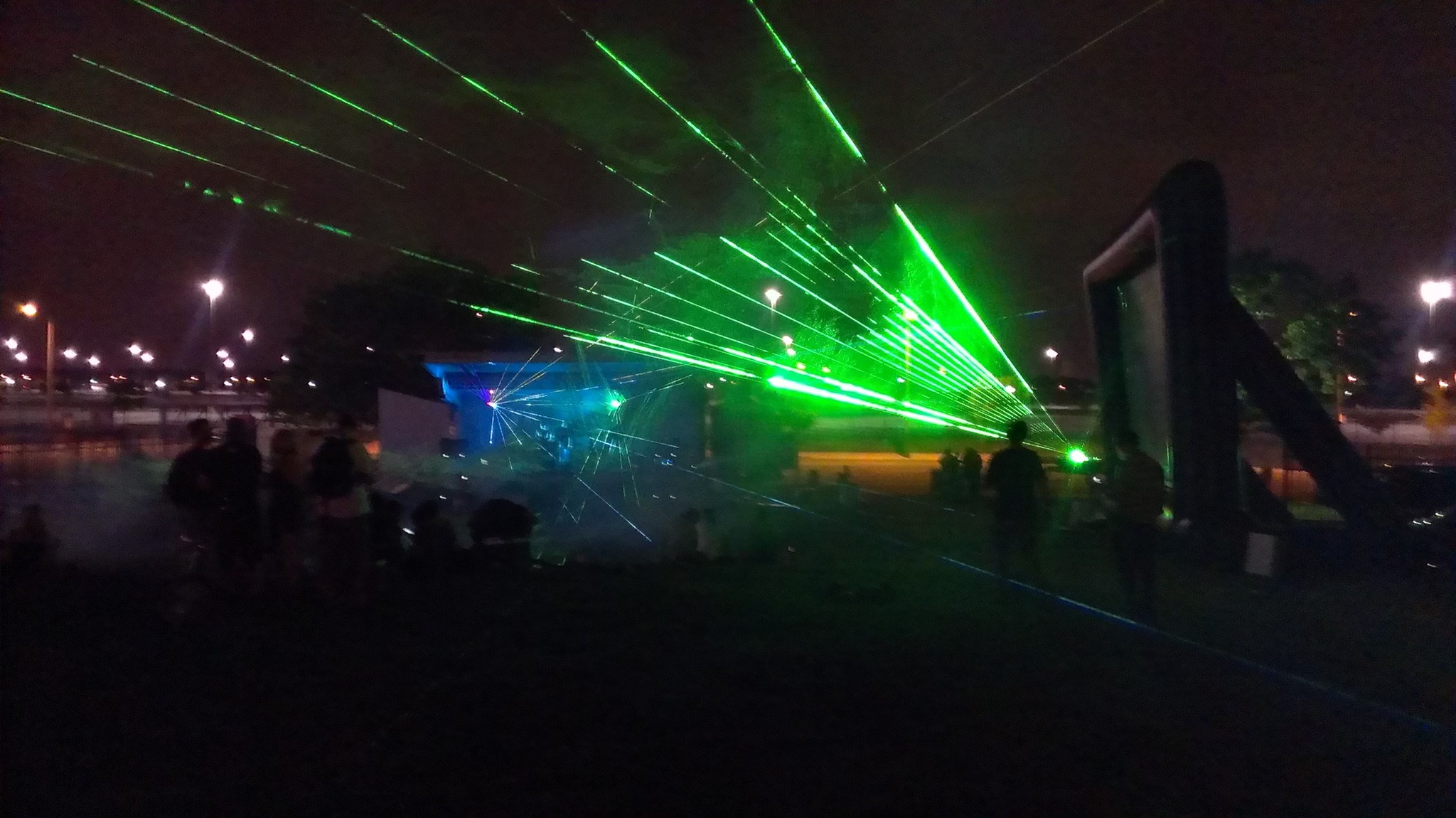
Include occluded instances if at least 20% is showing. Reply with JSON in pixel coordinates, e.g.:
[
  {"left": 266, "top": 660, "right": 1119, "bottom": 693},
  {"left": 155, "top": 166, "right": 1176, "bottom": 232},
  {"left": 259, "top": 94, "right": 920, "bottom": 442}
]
[
  {"left": 981, "top": 421, "right": 1050, "bottom": 584},
  {"left": 1084, "top": 426, "right": 1164, "bottom": 628},
  {"left": 161, "top": 404, "right": 859, "bottom": 580},
  {"left": 0, "top": 495, "right": 55, "bottom": 577},
  {"left": 937, "top": 449, "right": 982, "bottom": 502}
]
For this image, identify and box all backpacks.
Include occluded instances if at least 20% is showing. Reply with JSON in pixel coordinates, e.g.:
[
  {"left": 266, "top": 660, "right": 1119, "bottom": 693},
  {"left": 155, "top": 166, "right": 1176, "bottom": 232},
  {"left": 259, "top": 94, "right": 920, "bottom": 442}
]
[{"left": 309, "top": 437, "right": 358, "bottom": 498}]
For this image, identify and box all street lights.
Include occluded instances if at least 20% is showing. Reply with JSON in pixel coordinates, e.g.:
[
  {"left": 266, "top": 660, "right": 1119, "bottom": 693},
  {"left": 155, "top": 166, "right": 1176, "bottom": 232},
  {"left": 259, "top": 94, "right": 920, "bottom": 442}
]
[
  {"left": 20, "top": 302, "right": 54, "bottom": 410},
  {"left": 201, "top": 278, "right": 223, "bottom": 414}
]
